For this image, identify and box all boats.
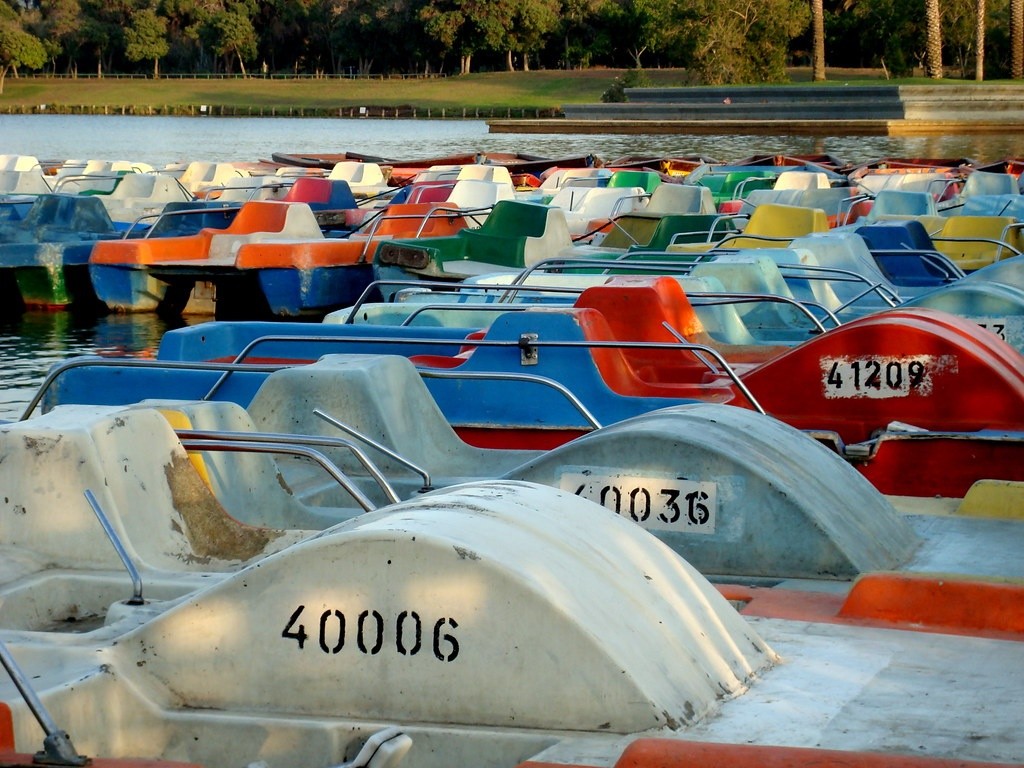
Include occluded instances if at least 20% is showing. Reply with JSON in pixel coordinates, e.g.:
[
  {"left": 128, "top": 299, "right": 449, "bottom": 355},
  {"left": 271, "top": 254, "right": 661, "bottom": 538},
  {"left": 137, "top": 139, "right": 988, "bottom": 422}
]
[{"left": 0, "top": 150, "right": 1023, "bottom": 768}]
